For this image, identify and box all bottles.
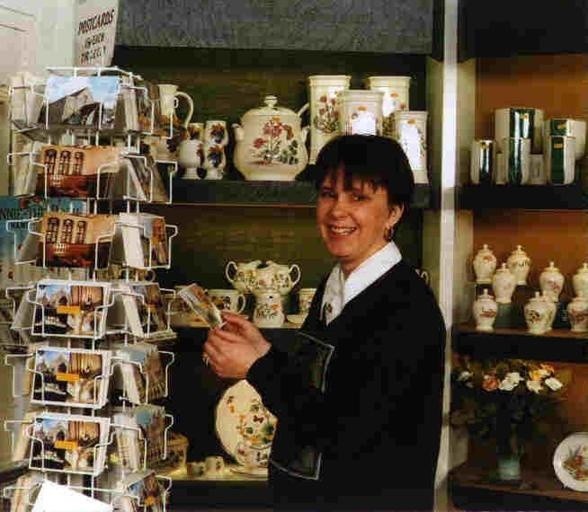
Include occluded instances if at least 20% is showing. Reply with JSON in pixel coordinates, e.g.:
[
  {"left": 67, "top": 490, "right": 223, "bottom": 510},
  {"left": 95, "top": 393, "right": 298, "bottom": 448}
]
[
  {"left": 153, "top": 84, "right": 194, "bottom": 160},
  {"left": 252, "top": 288, "right": 285, "bottom": 328},
  {"left": 472, "top": 242, "right": 588, "bottom": 336}
]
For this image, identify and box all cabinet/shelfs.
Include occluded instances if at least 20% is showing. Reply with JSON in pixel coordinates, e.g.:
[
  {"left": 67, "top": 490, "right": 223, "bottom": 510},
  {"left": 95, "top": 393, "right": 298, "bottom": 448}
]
[{"left": 72, "top": 0, "right": 588, "bottom": 512}]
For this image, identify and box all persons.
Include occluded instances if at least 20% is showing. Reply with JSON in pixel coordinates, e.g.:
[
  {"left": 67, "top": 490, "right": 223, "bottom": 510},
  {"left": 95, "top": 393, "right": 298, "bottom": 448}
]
[{"left": 201, "top": 131, "right": 447, "bottom": 510}]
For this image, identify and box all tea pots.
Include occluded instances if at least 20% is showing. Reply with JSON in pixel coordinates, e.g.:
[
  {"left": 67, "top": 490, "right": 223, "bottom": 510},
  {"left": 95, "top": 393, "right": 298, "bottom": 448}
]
[{"left": 232, "top": 95, "right": 309, "bottom": 181}]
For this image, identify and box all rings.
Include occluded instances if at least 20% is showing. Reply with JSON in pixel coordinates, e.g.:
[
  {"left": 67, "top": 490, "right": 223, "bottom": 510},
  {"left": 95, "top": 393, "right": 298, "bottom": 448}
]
[{"left": 202, "top": 353, "right": 210, "bottom": 366}]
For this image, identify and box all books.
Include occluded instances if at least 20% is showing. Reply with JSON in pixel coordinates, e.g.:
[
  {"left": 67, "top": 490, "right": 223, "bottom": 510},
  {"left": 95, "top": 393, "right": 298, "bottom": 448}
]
[
  {"left": 15, "top": 213, "right": 171, "bottom": 271},
  {"left": 8, "top": 279, "right": 174, "bottom": 341},
  {"left": 23, "top": 344, "right": 169, "bottom": 416},
  {"left": 11, "top": 403, "right": 169, "bottom": 478},
  {"left": 175, "top": 282, "right": 230, "bottom": 336},
  {"left": 8, "top": 132, "right": 172, "bottom": 203},
  {"left": 36, "top": 73, "right": 177, "bottom": 139},
  {"left": 4, "top": 471, "right": 167, "bottom": 512}
]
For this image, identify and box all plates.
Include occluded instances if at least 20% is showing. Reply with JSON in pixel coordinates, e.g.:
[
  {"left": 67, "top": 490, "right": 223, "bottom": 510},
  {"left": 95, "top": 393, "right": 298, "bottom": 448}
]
[
  {"left": 213, "top": 379, "right": 278, "bottom": 462},
  {"left": 551, "top": 430, "right": 588, "bottom": 493}
]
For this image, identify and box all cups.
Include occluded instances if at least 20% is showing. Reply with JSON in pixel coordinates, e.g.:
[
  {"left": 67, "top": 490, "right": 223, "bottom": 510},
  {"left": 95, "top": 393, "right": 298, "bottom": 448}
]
[
  {"left": 178, "top": 121, "right": 229, "bottom": 180},
  {"left": 186, "top": 456, "right": 224, "bottom": 476},
  {"left": 470, "top": 108, "right": 586, "bottom": 186},
  {"left": 226, "top": 260, "right": 300, "bottom": 295},
  {"left": 206, "top": 286, "right": 247, "bottom": 319}
]
